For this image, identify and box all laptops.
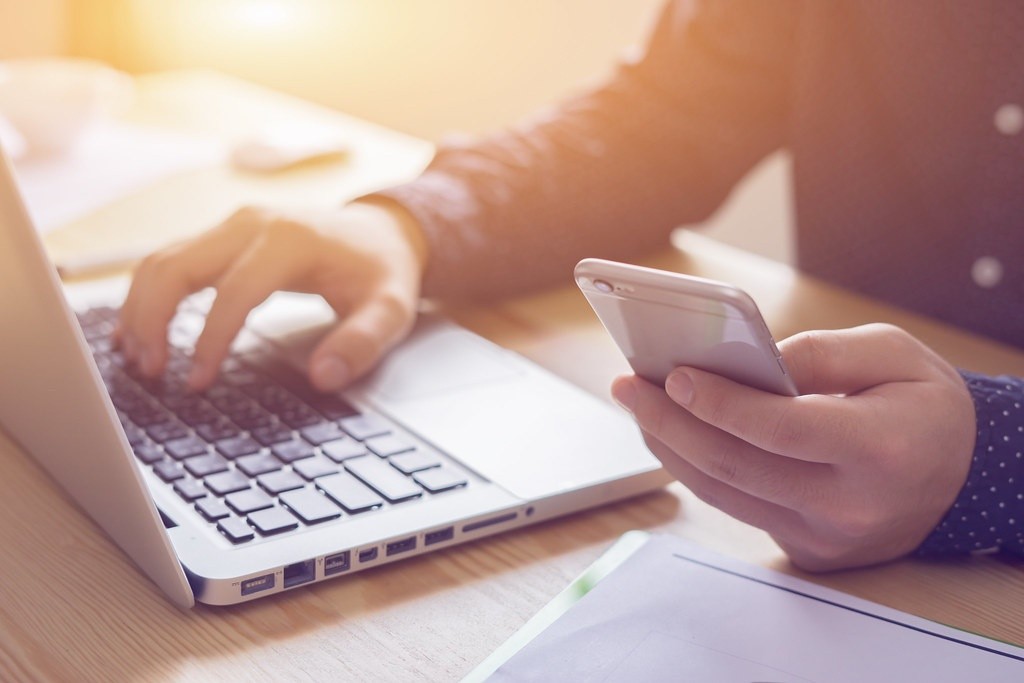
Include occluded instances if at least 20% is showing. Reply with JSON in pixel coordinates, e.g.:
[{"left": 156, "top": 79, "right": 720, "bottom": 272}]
[{"left": 0, "top": 136, "right": 676, "bottom": 607}]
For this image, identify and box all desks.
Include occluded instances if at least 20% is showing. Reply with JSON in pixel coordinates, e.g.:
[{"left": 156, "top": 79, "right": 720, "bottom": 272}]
[{"left": 0, "top": 152, "right": 1024, "bottom": 683}]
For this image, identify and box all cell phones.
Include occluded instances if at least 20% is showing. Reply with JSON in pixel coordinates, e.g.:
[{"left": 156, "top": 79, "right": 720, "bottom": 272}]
[{"left": 574, "top": 258, "right": 800, "bottom": 405}]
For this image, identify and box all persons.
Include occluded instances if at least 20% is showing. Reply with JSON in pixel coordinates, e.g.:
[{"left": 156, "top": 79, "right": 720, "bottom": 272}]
[{"left": 106, "top": 0, "right": 1024, "bottom": 577}]
